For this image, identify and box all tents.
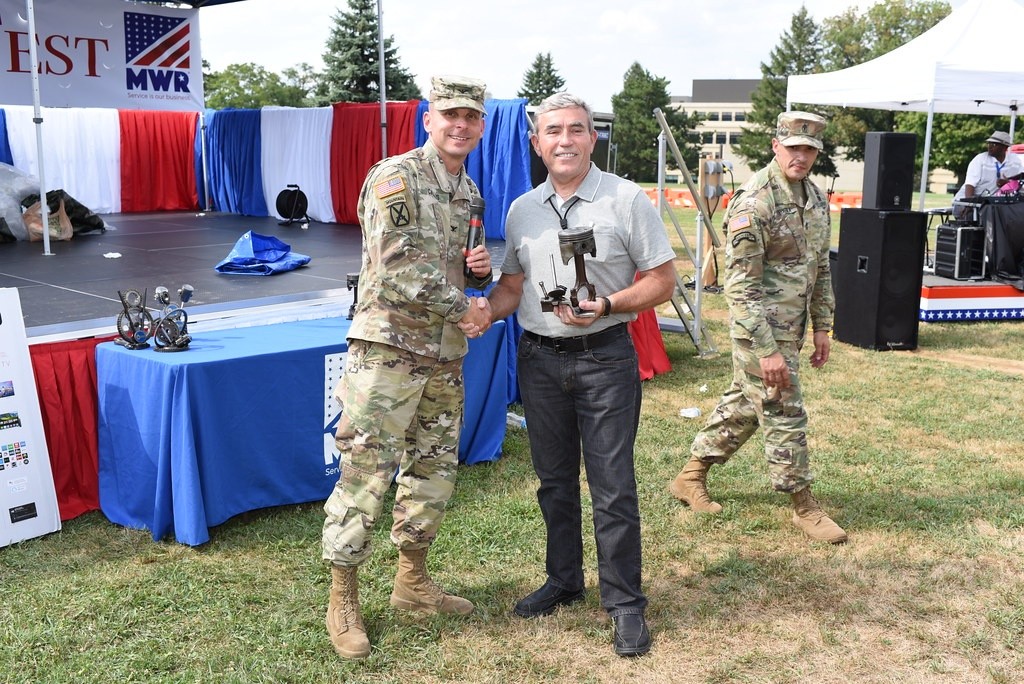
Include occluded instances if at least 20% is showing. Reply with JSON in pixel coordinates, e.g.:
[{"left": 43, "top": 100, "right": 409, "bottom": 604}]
[{"left": 786, "top": 0, "right": 1024, "bottom": 211}]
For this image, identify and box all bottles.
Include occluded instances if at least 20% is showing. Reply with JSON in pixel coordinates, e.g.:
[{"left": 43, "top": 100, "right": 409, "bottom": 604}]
[
  {"left": 506, "top": 413, "right": 527, "bottom": 429},
  {"left": 680, "top": 408, "right": 701, "bottom": 418}
]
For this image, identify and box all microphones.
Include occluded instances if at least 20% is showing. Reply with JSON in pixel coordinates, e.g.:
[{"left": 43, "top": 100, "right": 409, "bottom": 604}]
[{"left": 464, "top": 197, "right": 485, "bottom": 279}]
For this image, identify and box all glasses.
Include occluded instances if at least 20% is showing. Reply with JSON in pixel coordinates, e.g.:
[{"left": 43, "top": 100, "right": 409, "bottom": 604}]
[{"left": 987, "top": 142, "right": 1002, "bottom": 147}]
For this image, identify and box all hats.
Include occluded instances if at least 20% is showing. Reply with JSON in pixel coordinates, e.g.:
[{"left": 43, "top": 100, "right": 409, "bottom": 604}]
[
  {"left": 776, "top": 111, "right": 826, "bottom": 149},
  {"left": 429, "top": 72, "right": 488, "bottom": 116},
  {"left": 986, "top": 131, "right": 1010, "bottom": 147}
]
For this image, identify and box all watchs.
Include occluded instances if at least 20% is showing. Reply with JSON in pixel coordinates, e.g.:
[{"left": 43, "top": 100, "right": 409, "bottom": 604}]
[{"left": 596, "top": 296, "right": 611, "bottom": 318}]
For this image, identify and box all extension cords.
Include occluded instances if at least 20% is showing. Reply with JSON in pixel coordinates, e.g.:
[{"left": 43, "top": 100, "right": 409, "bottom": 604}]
[{"left": 923, "top": 266, "right": 935, "bottom": 273}]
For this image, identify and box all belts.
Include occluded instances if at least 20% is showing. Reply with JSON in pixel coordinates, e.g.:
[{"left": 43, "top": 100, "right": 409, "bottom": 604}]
[{"left": 524, "top": 322, "right": 628, "bottom": 353}]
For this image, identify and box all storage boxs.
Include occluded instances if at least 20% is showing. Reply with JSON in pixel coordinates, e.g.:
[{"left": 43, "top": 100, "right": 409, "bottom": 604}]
[{"left": 526, "top": 106, "right": 615, "bottom": 188}]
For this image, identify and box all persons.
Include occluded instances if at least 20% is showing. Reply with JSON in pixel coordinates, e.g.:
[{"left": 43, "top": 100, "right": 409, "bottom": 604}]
[
  {"left": 952, "top": 130, "right": 1024, "bottom": 219},
  {"left": 669, "top": 111, "right": 847, "bottom": 543},
  {"left": 321, "top": 75, "right": 493, "bottom": 660},
  {"left": 458, "top": 92, "right": 677, "bottom": 655}
]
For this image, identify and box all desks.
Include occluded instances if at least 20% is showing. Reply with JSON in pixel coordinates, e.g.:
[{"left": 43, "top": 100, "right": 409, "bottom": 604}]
[
  {"left": 954, "top": 201, "right": 1024, "bottom": 280},
  {"left": 96, "top": 316, "right": 506, "bottom": 546}
]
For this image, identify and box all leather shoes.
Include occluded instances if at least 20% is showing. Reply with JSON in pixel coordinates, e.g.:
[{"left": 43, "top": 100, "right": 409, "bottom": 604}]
[
  {"left": 514, "top": 577, "right": 585, "bottom": 619},
  {"left": 615, "top": 614, "right": 651, "bottom": 656}
]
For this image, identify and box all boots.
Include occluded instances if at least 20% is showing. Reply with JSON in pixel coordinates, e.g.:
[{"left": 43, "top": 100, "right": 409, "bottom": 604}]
[
  {"left": 790, "top": 485, "right": 847, "bottom": 544},
  {"left": 325, "top": 562, "right": 371, "bottom": 659},
  {"left": 670, "top": 454, "right": 723, "bottom": 514},
  {"left": 390, "top": 548, "right": 473, "bottom": 617}
]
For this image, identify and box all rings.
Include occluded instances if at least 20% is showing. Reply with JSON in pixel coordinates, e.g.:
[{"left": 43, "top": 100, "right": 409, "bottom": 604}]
[
  {"left": 484, "top": 259, "right": 488, "bottom": 267},
  {"left": 478, "top": 331, "right": 484, "bottom": 337}
]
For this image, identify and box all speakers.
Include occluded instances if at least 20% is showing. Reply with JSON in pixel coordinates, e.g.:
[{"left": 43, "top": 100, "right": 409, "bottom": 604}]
[{"left": 830, "top": 132, "right": 927, "bottom": 351}]
